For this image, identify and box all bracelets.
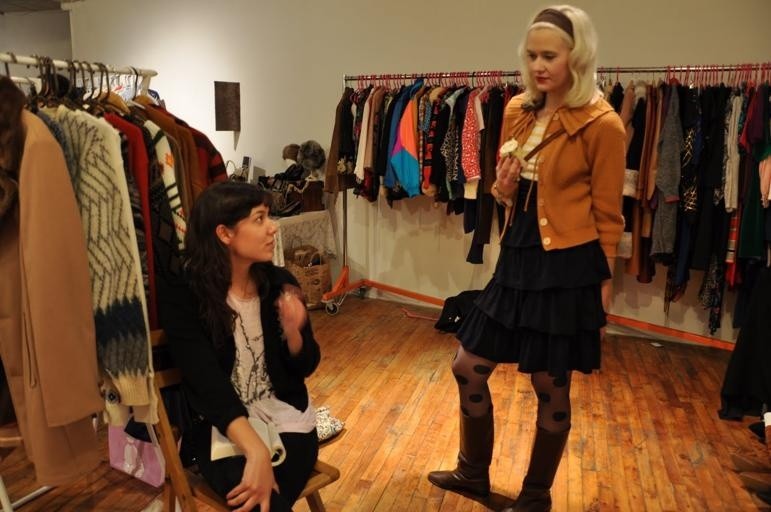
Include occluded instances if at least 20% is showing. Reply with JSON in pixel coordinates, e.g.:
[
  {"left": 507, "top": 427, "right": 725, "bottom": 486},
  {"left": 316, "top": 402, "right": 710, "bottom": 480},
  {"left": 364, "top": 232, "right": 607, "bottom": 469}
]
[{"left": 494, "top": 182, "right": 513, "bottom": 205}]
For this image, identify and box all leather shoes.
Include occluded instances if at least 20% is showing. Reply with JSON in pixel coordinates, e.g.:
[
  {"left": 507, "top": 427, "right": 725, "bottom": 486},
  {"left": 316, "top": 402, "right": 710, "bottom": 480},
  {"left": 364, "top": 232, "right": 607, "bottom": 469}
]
[{"left": 278, "top": 164, "right": 303, "bottom": 181}]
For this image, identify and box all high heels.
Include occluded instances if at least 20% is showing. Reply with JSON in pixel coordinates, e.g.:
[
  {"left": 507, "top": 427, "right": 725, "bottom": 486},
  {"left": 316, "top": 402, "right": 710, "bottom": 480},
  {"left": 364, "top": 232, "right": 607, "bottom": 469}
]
[{"left": 276, "top": 199, "right": 300, "bottom": 216}]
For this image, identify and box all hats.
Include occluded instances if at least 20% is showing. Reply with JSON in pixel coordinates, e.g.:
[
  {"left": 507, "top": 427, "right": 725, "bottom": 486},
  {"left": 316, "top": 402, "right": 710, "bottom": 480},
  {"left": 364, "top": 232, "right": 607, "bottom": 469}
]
[
  {"left": 296, "top": 140, "right": 325, "bottom": 170},
  {"left": 282, "top": 144, "right": 299, "bottom": 162}
]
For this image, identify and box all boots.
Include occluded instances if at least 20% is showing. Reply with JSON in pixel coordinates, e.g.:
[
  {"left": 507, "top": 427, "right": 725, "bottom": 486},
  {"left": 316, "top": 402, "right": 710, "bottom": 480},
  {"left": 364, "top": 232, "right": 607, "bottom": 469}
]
[
  {"left": 731, "top": 425, "right": 770, "bottom": 491},
  {"left": 428, "top": 406, "right": 493, "bottom": 502},
  {"left": 501, "top": 421, "right": 571, "bottom": 512}
]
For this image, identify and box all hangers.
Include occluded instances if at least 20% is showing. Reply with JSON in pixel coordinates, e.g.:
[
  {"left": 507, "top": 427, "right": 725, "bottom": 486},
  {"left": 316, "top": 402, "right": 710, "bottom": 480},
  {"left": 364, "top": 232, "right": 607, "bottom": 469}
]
[
  {"left": 5, "top": 51, "right": 146, "bottom": 123},
  {"left": 346, "top": 63, "right": 525, "bottom": 102},
  {"left": 596, "top": 61, "right": 771, "bottom": 104}
]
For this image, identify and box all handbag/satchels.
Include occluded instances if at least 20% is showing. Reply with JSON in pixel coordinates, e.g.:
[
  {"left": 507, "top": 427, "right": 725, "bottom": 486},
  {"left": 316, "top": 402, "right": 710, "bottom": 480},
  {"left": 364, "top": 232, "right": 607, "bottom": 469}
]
[
  {"left": 263, "top": 190, "right": 284, "bottom": 213},
  {"left": 108, "top": 422, "right": 183, "bottom": 488},
  {"left": 433, "top": 290, "right": 480, "bottom": 332},
  {"left": 284, "top": 244, "right": 330, "bottom": 310}
]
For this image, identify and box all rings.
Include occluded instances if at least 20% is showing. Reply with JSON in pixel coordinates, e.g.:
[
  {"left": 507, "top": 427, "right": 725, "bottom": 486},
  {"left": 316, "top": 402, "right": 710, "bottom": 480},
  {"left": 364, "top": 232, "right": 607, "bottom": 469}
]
[{"left": 514, "top": 177, "right": 520, "bottom": 182}]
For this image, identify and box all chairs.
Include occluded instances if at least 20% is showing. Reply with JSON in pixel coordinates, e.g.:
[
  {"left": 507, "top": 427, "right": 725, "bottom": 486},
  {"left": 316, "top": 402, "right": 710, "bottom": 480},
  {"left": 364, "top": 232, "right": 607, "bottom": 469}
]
[{"left": 145, "top": 330, "right": 340, "bottom": 512}]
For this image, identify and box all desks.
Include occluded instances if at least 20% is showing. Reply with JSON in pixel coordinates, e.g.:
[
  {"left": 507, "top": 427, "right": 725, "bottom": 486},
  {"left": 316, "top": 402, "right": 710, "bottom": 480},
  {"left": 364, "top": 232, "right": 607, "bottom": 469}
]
[{"left": 272, "top": 209, "right": 331, "bottom": 266}]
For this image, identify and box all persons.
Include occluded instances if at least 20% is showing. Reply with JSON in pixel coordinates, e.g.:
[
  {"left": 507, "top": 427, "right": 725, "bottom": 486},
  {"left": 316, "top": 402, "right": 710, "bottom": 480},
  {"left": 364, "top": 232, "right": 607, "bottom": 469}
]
[
  {"left": 161, "top": 180, "right": 321, "bottom": 511},
  {"left": 428, "top": 3, "right": 627, "bottom": 512}
]
